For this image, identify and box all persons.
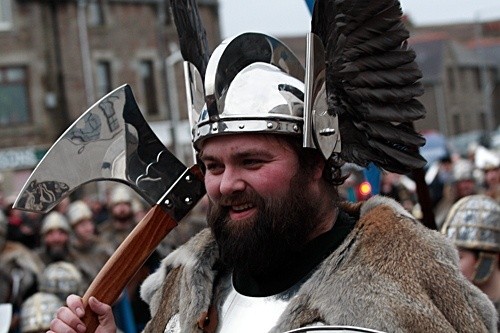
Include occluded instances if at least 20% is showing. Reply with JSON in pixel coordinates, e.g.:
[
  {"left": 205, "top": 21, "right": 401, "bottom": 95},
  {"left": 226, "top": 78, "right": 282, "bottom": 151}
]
[
  {"left": 357, "top": 120, "right": 498, "bottom": 211},
  {"left": 1, "top": 188, "right": 158, "bottom": 332},
  {"left": 43, "top": 66, "right": 497, "bottom": 333},
  {"left": 441, "top": 194, "right": 500, "bottom": 333}
]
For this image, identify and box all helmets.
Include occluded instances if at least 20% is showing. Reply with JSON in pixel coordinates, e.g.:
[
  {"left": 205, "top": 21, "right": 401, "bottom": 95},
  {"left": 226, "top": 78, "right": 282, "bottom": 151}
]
[
  {"left": 4, "top": 193, "right": 19, "bottom": 210},
  {"left": 40, "top": 262, "right": 81, "bottom": 299},
  {"left": 19, "top": 292, "right": 62, "bottom": 332},
  {"left": 474, "top": 146, "right": 500, "bottom": 171},
  {"left": 438, "top": 194, "right": 500, "bottom": 284},
  {"left": 67, "top": 200, "right": 93, "bottom": 226},
  {"left": 169, "top": 0, "right": 429, "bottom": 186},
  {"left": 40, "top": 211, "right": 70, "bottom": 235},
  {"left": 108, "top": 183, "right": 133, "bottom": 208},
  {"left": 453, "top": 160, "right": 475, "bottom": 183}
]
